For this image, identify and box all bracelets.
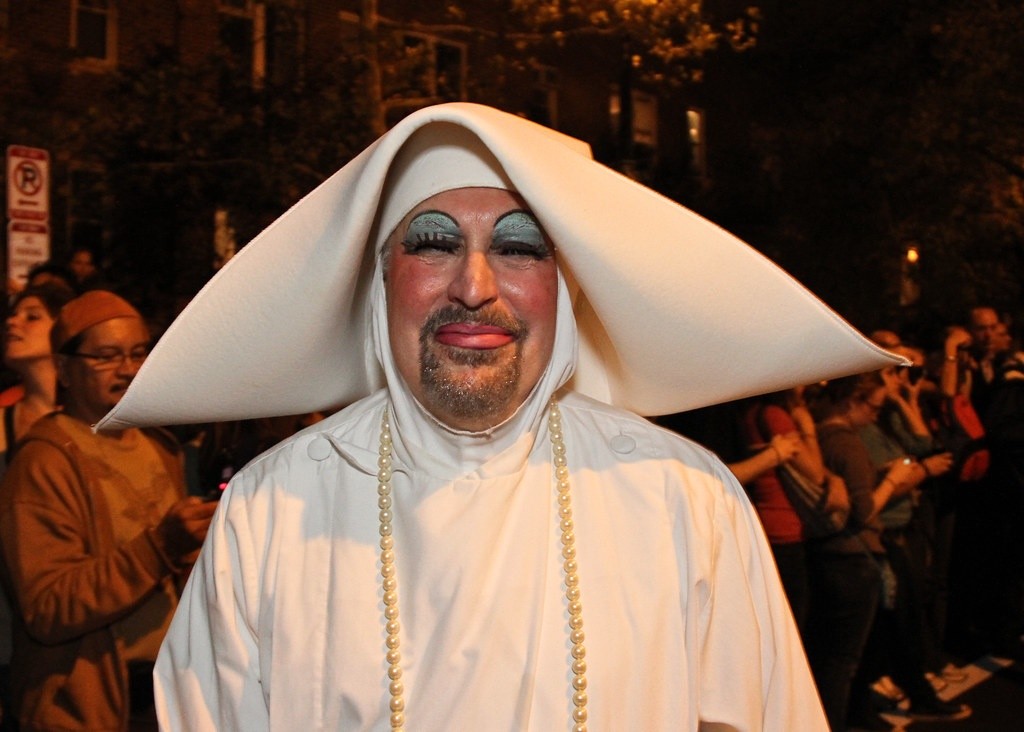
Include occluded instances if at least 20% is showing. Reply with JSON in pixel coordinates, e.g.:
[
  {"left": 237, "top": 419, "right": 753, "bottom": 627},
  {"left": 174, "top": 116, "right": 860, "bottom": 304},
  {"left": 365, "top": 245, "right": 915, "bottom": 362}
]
[
  {"left": 885, "top": 477, "right": 897, "bottom": 489},
  {"left": 945, "top": 355, "right": 957, "bottom": 361},
  {"left": 770, "top": 442, "right": 783, "bottom": 464},
  {"left": 920, "top": 460, "right": 929, "bottom": 475}
]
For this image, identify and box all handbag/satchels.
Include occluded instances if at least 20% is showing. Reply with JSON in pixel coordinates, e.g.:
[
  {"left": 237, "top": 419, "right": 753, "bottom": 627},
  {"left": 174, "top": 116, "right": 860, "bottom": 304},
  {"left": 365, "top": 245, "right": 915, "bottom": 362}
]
[
  {"left": 880, "top": 560, "right": 896, "bottom": 608},
  {"left": 759, "top": 410, "right": 849, "bottom": 536}
]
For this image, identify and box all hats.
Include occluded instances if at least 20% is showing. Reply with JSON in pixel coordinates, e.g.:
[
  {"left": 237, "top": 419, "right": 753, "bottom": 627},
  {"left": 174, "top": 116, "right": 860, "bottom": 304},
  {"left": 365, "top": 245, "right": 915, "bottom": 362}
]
[{"left": 47, "top": 290, "right": 141, "bottom": 406}]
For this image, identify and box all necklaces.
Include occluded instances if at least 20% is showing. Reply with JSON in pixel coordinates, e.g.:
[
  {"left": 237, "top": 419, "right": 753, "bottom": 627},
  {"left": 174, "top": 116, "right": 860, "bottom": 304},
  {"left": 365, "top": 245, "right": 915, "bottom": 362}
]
[{"left": 378, "top": 392, "right": 589, "bottom": 732}]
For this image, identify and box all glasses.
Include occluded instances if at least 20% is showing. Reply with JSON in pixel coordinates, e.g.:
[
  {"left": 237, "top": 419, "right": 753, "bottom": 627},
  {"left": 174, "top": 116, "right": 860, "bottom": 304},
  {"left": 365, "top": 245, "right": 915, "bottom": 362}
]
[
  {"left": 72, "top": 346, "right": 147, "bottom": 368},
  {"left": 864, "top": 401, "right": 879, "bottom": 413}
]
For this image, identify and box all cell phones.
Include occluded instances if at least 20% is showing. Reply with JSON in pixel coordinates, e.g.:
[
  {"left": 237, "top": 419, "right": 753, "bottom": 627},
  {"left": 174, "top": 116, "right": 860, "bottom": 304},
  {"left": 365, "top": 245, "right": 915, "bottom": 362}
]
[
  {"left": 202, "top": 478, "right": 230, "bottom": 503},
  {"left": 962, "top": 336, "right": 985, "bottom": 363}
]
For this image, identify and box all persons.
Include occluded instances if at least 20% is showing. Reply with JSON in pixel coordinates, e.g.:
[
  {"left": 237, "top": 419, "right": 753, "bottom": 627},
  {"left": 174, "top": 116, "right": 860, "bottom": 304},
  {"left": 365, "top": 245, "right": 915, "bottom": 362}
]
[
  {"left": 93, "top": 104, "right": 910, "bottom": 732},
  {"left": 709, "top": 307, "right": 1024, "bottom": 732},
  {"left": 0, "top": 250, "right": 347, "bottom": 732}
]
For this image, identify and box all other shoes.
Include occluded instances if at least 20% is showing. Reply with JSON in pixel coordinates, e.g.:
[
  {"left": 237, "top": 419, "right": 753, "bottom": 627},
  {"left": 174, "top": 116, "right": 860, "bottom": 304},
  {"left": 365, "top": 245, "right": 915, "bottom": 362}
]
[
  {"left": 909, "top": 695, "right": 971, "bottom": 722},
  {"left": 944, "top": 663, "right": 966, "bottom": 682},
  {"left": 926, "top": 673, "right": 948, "bottom": 691}
]
